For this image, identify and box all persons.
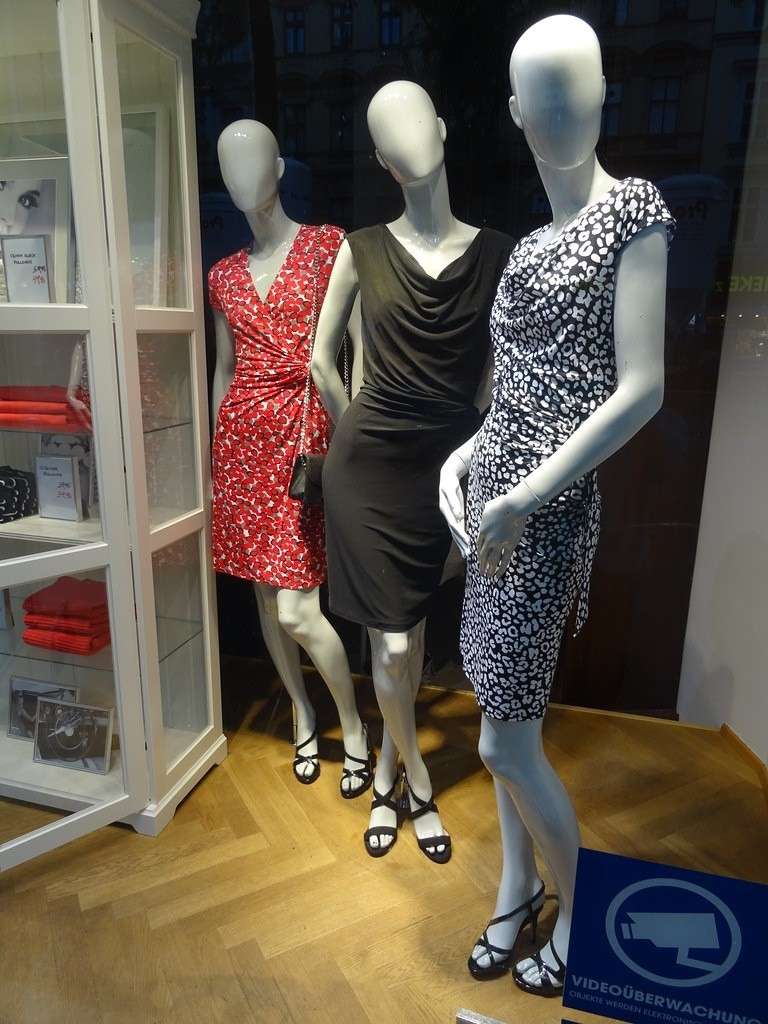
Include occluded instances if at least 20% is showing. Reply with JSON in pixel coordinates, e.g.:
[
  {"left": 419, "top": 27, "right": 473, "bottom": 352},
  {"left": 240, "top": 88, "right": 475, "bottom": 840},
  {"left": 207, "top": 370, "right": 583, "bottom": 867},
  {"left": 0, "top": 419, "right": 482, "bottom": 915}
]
[
  {"left": 207, "top": 119, "right": 364, "bottom": 800},
  {"left": 311, "top": 80, "right": 516, "bottom": 858},
  {"left": 437, "top": 12, "right": 678, "bottom": 986},
  {"left": 11, "top": 686, "right": 37, "bottom": 737}
]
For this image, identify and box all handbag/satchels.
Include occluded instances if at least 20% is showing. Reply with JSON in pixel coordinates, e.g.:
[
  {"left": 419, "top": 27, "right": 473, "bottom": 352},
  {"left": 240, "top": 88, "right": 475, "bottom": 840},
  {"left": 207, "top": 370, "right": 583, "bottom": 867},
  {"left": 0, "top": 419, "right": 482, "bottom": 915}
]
[{"left": 287, "top": 455, "right": 328, "bottom": 503}]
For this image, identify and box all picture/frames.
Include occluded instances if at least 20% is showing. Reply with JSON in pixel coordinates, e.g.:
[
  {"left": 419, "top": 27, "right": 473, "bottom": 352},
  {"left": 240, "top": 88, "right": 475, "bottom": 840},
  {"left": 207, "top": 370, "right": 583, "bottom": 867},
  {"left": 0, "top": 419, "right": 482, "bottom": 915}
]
[
  {"left": 0, "top": 101, "right": 171, "bottom": 312},
  {"left": 6, "top": 675, "right": 79, "bottom": 743},
  {"left": 32, "top": 697, "right": 113, "bottom": 776},
  {"left": 0, "top": 156, "right": 73, "bottom": 305},
  {"left": 37, "top": 432, "right": 93, "bottom": 510}
]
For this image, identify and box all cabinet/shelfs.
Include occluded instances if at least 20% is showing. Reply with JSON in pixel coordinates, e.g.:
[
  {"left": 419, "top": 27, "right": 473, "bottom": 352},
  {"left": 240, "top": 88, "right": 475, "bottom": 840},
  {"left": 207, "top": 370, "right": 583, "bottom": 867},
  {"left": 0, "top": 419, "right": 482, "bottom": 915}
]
[{"left": 0, "top": 0, "right": 228, "bottom": 835}]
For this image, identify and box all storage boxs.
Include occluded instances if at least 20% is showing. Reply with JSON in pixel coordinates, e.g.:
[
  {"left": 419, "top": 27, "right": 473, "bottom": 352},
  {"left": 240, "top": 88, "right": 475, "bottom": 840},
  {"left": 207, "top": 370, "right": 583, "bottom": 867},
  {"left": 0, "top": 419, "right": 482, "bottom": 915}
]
[{"left": 34, "top": 455, "right": 83, "bottom": 521}]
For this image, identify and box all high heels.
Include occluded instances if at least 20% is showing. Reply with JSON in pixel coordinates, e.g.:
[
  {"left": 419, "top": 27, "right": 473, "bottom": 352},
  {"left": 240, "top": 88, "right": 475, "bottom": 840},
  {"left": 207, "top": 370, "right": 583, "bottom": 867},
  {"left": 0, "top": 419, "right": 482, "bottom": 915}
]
[
  {"left": 293, "top": 716, "right": 320, "bottom": 785},
  {"left": 467, "top": 878, "right": 547, "bottom": 980},
  {"left": 401, "top": 766, "right": 451, "bottom": 864},
  {"left": 339, "top": 722, "right": 373, "bottom": 799},
  {"left": 363, "top": 775, "right": 399, "bottom": 856},
  {"left": 512, "top": 938, "right": 567, "bottom": 997}
]
